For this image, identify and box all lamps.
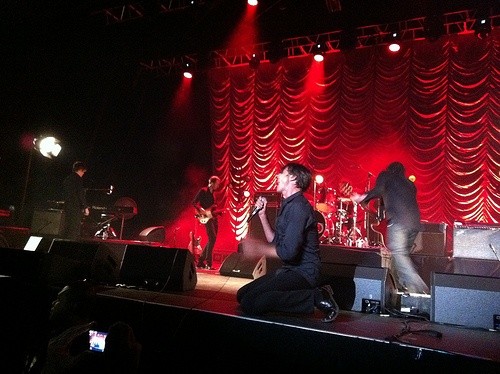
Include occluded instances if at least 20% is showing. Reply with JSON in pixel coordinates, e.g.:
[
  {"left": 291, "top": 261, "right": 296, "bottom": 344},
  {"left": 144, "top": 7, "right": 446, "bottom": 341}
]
[
  {"left": 33, "top": 135, "right": 61, "bottom": 159},
  {"left": 314, "top": 43, "right": 325, "bottom": 62},
  {"left": 183, "top": 61, "right": 194, "bottom": 78},
  {"left": 389, "top": 33, "right": 401, "bottom": 52},
  {"left": 250, "top": 53, "right": 261, "bottom": 68}
]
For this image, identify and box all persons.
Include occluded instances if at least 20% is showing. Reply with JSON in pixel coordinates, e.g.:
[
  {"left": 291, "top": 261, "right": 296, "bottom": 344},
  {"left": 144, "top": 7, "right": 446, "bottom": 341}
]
[
  {"left": 236, "top": 163, "right": 338, "bottom": 322},
  {"left": 62, "top": 161, "right": 89, "bottom": 239},
  {"left": 23, "top": 283, "right": 142, "bottom": 374},
  {"left": 192, "top": 176, "right": 225, "bottom": 270},
  {"left": 350, "top": 162, "right": 429, "bottom": 294}
]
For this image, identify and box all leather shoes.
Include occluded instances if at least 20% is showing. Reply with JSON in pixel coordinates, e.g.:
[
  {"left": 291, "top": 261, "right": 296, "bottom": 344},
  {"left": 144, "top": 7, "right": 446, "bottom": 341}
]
[{"left": 316, "top": 285, "right": 340, "bottom": 322}]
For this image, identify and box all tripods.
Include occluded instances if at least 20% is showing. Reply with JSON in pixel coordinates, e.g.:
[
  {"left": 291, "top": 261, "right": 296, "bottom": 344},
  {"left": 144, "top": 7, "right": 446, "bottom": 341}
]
[{"left": 328, "top": 200, "right": 368, "bottom": 247}]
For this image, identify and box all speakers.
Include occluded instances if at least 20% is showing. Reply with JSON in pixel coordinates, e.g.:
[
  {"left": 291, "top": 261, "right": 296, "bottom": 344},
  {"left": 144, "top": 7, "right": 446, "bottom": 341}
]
[
  {"left": 139, "top": 226, "right": 165, "bottom": 243},
  {"left": 47, "top": 239, "right": 121, "bottom": 288},
  {"left": 217, "top": 240, "right": 278, "bottom": 278},
  {"left": 409, "top": 229, "right": 447, "bottom": 256},
  {"left": 31, "top": 208, "right": 66, "bottom": 237},
  {"left": 118, "top": 243, "right": 198, "bottom": 291},
  {"left": 317, "top": 264, "right": 396, "bottom": 313},
  {"left": 452, "top": 225, "right": 500, "bottom": 261},
  {"left": 430, "top": 272, "right": 500, "bottom": 332}
]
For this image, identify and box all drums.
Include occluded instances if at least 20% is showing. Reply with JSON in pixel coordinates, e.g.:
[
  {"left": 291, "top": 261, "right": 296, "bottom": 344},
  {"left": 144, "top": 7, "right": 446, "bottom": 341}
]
[
  {"left": 314, "top": 209, "right": 335, "bottom": 243},
  {"left": 335, "top": 197, "right": 352, "bottom": 217},
  {"left": 335, "top": 218, "right": 356, "bottom": 244},
  {"left": 316, "top": 188, "right": 337, "bottom": 212}
]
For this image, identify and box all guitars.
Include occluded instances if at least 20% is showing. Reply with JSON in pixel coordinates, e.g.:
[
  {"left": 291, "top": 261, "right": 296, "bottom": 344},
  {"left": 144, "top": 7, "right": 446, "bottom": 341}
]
[
  {"left": 339, "top": 181, "right": 389, "bottom": 250},
  {"left": 187, "top": 218, "right": 203, "bottom": 255},
  {"left": 195, "top": 204, "right": 243, "bottom": 225}
]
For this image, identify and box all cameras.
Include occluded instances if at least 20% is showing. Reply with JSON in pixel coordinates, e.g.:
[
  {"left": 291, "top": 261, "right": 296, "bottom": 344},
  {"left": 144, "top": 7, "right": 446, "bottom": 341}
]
[{"left": 88, "top": 327, "right": 111, "bottom": 353}]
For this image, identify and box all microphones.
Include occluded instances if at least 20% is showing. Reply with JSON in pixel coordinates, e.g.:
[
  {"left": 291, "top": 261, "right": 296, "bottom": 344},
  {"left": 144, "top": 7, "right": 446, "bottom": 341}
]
[{"left": 246, "top": 196, "right": 266, "bottom": 223}]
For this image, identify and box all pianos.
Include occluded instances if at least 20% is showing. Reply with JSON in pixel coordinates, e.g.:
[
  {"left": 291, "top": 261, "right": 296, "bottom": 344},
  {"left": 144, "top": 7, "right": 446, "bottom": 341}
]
[{"left": 79, "top": 205, "right": 133, "bottom": 240}]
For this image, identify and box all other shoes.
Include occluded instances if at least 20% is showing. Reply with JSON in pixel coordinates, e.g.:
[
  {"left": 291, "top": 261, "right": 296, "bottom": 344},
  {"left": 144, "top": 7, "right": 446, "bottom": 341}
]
[
  {"left": 205, "top": 264, "right": 216, "bottom": 270},
  {"left": 196, "top": 263, "right": 205, "bottom": 269}
]
